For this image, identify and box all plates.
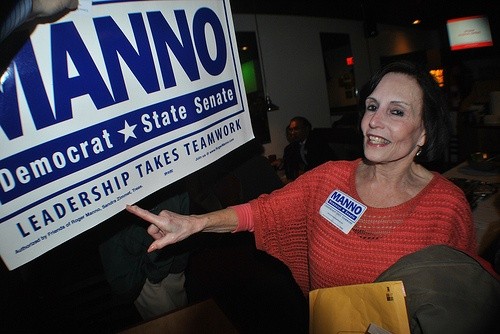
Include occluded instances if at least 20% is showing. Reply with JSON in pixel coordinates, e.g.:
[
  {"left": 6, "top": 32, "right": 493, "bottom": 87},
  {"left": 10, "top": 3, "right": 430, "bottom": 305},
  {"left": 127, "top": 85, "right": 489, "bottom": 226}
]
[{"left": 452, "top": 178, "right": 498, "bottom": 210}]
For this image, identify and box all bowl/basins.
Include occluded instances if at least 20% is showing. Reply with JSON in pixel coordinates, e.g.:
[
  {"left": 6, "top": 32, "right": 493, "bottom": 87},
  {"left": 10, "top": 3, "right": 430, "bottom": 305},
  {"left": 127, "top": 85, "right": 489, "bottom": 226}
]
[{"left": 468, "top": 151, "right": 500, "bottom": 171}]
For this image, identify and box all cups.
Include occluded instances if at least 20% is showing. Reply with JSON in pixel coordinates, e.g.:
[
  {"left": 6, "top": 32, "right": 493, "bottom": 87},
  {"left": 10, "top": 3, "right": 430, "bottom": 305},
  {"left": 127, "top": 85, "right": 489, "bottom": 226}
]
[{"left": 278, "top": 170, "right": 286, "bottom": 181}]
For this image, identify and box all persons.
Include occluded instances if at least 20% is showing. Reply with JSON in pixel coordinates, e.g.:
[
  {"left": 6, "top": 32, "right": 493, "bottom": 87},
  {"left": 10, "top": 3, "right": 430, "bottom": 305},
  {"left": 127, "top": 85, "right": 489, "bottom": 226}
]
[
  {"left": 126, "top": 63, "right": 478, "bottom": 334},
  {"left": 130, "top": 182, "right": 192, "bottom": 321},
  {"left": 270, "top": 117, "right": 327, "bottom": 183}
]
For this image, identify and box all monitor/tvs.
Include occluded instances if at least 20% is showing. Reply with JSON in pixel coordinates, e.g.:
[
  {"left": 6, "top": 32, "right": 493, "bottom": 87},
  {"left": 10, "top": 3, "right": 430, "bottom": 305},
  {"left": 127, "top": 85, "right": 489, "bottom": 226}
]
[{"left": 444, "top": 14, "right": 495, "bottom": 52}]
